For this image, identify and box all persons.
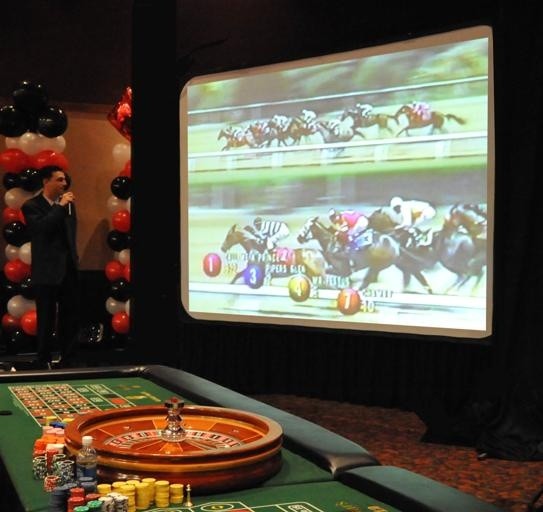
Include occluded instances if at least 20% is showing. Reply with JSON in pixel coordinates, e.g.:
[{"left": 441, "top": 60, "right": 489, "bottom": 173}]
[
  {"left": 244, "top": 197, "right": 489, "bottom": 260},
  {"left": 224, "top": 100, "right": 432, "bottom": 139},
  {"left": 20, "top": 163, "right": 80, "bottom": 368}
]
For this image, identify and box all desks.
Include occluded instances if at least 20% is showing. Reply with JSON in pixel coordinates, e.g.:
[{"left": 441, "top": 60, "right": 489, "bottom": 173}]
[{"left": 0, "top": 361, "right": 508, "bottom": 512}]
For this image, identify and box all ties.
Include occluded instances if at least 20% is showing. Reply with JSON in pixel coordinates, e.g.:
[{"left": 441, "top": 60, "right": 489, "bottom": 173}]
[{"left": 52, "top": 201, "right": 54, "bottom": 205}]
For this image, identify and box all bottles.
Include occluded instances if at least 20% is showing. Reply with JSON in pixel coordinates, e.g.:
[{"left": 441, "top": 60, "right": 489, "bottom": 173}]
[{"left": 76, "top": 436, "right": 97, "bottom": 480}]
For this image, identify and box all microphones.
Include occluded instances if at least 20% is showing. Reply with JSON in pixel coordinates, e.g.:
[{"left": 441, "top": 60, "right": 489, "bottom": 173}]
[{"left": 68, "top": 188, "right": 73, "bottom": 216}]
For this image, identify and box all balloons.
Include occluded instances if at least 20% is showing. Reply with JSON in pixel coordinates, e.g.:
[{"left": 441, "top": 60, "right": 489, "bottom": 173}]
[
  {"left": 0, "top": 76, "right": 71, "bottom": 192},
  {"left": 0, "top": 186, "right": 38, "bottom": 353},
  {"left": 105, "top": 145, "right": 130, "bottom": 334}
]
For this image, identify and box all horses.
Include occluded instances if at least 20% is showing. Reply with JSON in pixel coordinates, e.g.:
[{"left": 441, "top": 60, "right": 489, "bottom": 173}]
[
  {"left": 296, "top": 215, "right": 433, "bottom": 293},
  {"left": 367, "top": 204, "right": 487, "bottom": 296},
  {"left": 221, "top": 225, "right": 328, "bottom": 287},
  {"left": 394, "top": 105, "right": 467, "bottom": 139},
  {"left": 309, "top": 121, "right": 365, "bottom": 157},
  {"left": 219, "top": 115, "right": 318, "bottom": 152},
  {"left": 341, "top": 108, "right": 400, "bottom": 135}
]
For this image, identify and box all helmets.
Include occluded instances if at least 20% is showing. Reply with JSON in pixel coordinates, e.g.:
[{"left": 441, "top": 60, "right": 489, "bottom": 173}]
[
  {"left": 390, "top": 196, "right": 404, "bottom": 208},
  {"left": 328, "top": 208, "right": 340, "bottom": 222},
  {"left": 254, "top": 217, "right": 262, "bottom": 225}
]
